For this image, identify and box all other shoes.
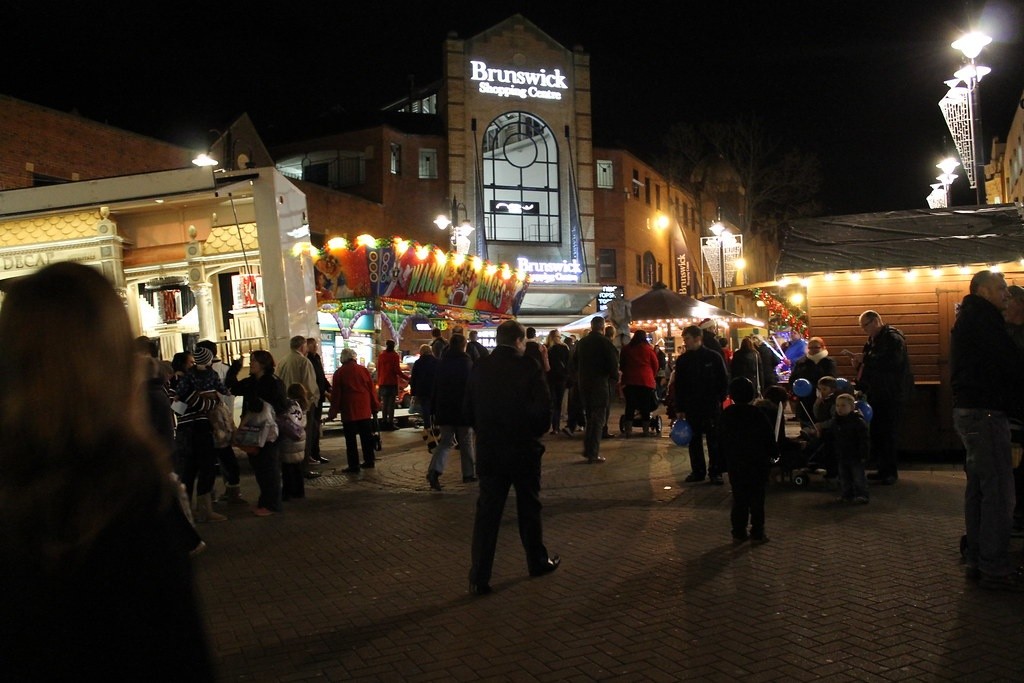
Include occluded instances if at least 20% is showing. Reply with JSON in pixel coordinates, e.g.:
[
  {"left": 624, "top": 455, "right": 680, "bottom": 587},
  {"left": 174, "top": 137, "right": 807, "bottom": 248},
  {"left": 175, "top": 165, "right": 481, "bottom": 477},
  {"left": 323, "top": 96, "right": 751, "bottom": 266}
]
[
  {"left": 710, "top": 476, "right": 724, "bottom": 484},
  {"left": 561, "top": 426, "right": 574, "bottom": 439},
  {"left": 965, "top": 561, "right": 1024, "bottom": 591},
  {"left": 342, "top": 466, "right": 360, "bottom": 472},
  {"left": 751, "top": 537, "right": 769, "bottom": 545},
  {"left": 866, "top": 469, "right": 897, "bottom": 483},
  {"left": 584, "top": 455, "right": 606, "bottom": 463},
  {"left": 382, "top": 422, "right": 399, "bottom": 430},
  {"left": 734, "top": 535, "right": 748, "bottom": 544},
  {"left": 312, "top": 456, "right": 329, "bottom": 463},
  {"left": 425, "top": 473, "right": 443, "bottom": 490},
  {"left": 686, "top": 471, "right": 706, "bottom": 482},
  {"left": 305, "top": 455, "right": 321, "bottom": 465},
  {"left": 360, "top": 461, "right": 375, "bottom": 468},
  {"left": 550, "top": 429, "right": 560, "bottom": 435},
  {"left": 303, "top": 470, "right": 320, "bottom": 478},
  {"left": 250, "top": 503, "right": 279, "bottom": 516},
  {"left": 462, "top": 476, "right": 477, "bottom": 482}
]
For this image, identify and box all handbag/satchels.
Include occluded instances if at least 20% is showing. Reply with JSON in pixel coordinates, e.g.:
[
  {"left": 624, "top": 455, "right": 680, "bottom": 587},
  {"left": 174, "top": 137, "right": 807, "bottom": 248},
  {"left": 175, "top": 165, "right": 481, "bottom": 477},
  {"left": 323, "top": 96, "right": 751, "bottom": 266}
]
[
  {"left": 423, "top": 428, "right": 458, "bottom": 454},
  {"left": 232, "top": 401, "right": 279, "bottom": 446},
  {"left": 374, "top": 434, "right": 382, "bottom": 451}
]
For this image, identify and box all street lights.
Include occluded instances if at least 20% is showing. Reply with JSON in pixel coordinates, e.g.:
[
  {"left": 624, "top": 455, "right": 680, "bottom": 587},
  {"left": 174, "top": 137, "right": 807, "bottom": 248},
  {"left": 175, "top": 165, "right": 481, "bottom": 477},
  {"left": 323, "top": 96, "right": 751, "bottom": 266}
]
[
  {"left": 711, "top": 221, "right": 730, "bottom": 310},
  {"left": 936, "top": 155, "right": 961, "bottom": 207},
  {"left": 951, "top": 15, "right": 993, "bottom": 207}
]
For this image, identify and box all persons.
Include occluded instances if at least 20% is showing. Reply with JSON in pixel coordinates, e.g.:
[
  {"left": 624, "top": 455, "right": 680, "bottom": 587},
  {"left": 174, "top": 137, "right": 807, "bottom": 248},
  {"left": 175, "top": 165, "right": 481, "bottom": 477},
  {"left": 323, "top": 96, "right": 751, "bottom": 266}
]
[
  {"left": 410, "top": 328, "right": 489, "bottom": 491},
  {"left": 714, "top": 377, "right": 781, "bottom": 546},
  {"left": 666, "top": 325, "right": 729, "bottom": 485},
  {"left": 465, "top": 320, "right": 562, "bottom": 596},
  {"left": 134, "top": 335, "right": 332, "bottom": 525},
  {"left": 948, "top": 270, "right": 1024, "bottom": 597},
  {"left": 524, "top": 316, "right": 615, "bottom": 465},
  {"left": 0, "top": 262, "right": 217, "bottom": 683},
  {"left": 325, "top": 349, "right": 381, "bottom": 473},
  {"left": 377, "top": 339, "right": 411, "bottom": 431},
  {"left": 703, "top": 331, "right": 870, "bottom": 505},
  {"left": 654, "top": 345, "right": 665, "bottom": 403},
  {"left": 619, "top": 330, "right": 659, "bottom": 440},
  {"left": 850, "top": 310, "right": 914, "bottom": 486}
]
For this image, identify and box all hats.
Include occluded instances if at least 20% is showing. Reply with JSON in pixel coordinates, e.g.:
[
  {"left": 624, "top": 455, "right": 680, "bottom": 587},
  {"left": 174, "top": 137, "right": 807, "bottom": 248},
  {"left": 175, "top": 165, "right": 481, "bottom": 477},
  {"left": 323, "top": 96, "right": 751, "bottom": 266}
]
[
  {"left": 193, "top": 346, "right": 213, "bottom": 366},
  {"left": 698, "top": 318, "right": 714, "bottom": 329},
  {"left": 339, "top": 347, "right": 357, "bottom": 362}
]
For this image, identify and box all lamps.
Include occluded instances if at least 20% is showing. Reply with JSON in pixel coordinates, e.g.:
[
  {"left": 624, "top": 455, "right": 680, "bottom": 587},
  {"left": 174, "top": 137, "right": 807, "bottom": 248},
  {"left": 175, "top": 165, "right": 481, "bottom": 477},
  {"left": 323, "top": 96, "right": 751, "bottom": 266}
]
[
  {"left": 929, "top": 265, "right": 945, "bottom": 279},
  {"left": 875, "top": 267, "right": 889, "bottom": 278},
  {"left": 824, "top": 268, "right": 836, "bottom": 281},
  {"left": 906, "top": 266, "right": 917, "bottom": 281},
  {"left": 849, "top": 270, "right": 862, "bottom": 282},
  {"left": 191, "top": 129, "right": 226, "bottom": 173},
  {"left": 986, "top": 262, "right": 1003, "bottom": 273},
  {"left": 957, "top": 263, "right": 972, "bottom": 276}
]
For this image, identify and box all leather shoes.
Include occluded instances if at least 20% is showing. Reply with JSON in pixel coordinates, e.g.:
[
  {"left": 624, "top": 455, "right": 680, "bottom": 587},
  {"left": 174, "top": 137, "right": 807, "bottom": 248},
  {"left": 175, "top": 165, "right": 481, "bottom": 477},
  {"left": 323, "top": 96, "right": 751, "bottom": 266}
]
[
  {"left": 469, "top": 581, "right": 492, "bottom": 593},
  {"left": 529, "top": 553, "right": 562, "bottom": 576}
]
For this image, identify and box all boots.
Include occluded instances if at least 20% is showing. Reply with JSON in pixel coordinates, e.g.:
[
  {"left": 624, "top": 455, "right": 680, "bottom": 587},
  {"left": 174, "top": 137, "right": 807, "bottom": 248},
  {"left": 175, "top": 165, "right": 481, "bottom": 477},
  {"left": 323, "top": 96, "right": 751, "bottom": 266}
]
[
  {"left": 223, "top": 481, "right": 249, "bottom": 505},
  {"left": 194, "top": 492, "right": 227, "bottom": 522}
]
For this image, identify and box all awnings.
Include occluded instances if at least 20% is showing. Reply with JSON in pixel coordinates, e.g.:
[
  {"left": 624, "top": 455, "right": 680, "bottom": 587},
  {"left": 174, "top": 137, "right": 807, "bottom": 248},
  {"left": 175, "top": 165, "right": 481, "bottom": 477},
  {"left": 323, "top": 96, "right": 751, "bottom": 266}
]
[{"left": 427, "top": 286, "right": 602, "bottom": 326}]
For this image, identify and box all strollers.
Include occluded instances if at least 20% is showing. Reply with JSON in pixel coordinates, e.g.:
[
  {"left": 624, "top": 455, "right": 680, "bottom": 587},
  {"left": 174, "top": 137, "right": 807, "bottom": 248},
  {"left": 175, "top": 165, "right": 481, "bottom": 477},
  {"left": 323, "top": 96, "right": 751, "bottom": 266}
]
[
  {"left": 620, "top": 381, "right": 662, "bottom": 434},
  {"left": 774, "top": 384, "right": 865, "bottom": 486}
]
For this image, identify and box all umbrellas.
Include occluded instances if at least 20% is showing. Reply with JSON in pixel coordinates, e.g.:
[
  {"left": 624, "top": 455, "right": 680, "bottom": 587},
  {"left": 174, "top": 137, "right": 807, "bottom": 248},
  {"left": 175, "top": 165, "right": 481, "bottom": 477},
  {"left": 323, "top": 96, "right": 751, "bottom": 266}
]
[{"left": 558, "top": 281, "right": 743, "bottom": 362}]
